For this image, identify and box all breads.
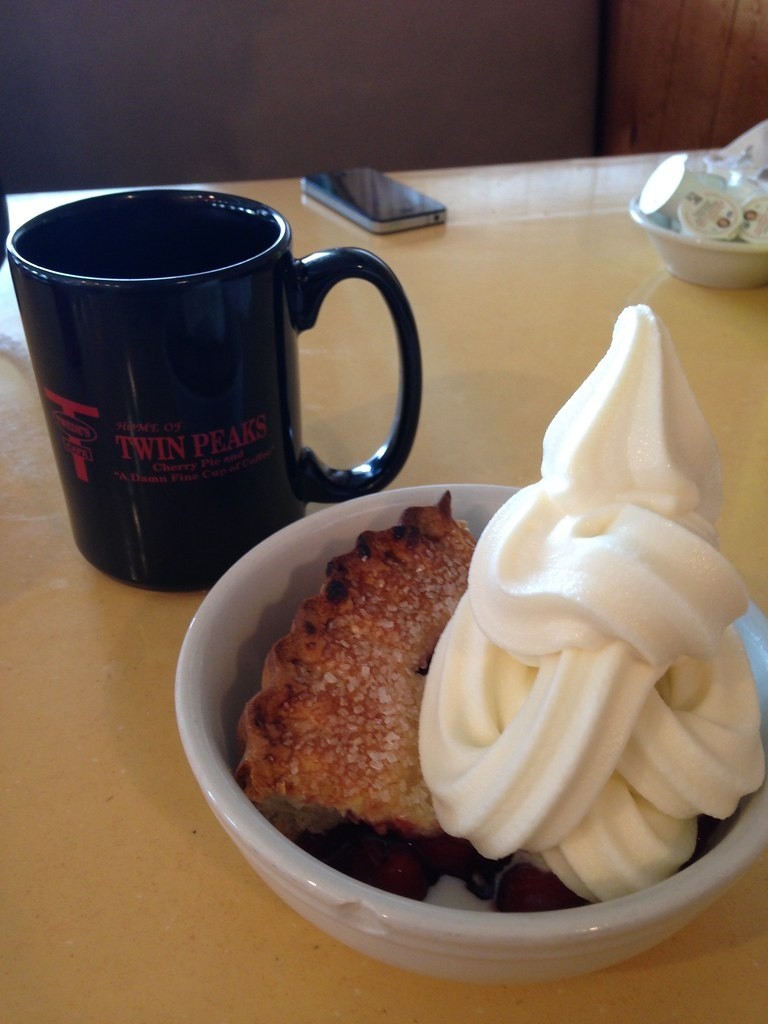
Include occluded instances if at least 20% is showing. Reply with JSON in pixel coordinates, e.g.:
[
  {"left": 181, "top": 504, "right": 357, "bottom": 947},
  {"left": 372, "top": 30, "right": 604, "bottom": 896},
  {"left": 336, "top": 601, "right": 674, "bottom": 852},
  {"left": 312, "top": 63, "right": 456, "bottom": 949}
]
[{"left": 239, "top": 494, "right": 491, "bottom": 841}]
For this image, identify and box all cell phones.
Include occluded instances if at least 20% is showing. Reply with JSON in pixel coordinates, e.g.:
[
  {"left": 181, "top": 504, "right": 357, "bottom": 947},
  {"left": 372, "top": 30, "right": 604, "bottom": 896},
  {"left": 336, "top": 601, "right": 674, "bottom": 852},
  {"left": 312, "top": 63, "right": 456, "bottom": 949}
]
[{"left": 301, "top": 167, "right": 448, "bottom": 235}]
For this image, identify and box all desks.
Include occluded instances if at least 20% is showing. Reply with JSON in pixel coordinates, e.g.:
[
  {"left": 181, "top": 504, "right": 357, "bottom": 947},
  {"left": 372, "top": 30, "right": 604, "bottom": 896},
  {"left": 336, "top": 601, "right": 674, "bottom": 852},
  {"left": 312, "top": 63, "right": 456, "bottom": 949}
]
[{"left": 0, "top": 151, "right": 768, "bottom": 1024}]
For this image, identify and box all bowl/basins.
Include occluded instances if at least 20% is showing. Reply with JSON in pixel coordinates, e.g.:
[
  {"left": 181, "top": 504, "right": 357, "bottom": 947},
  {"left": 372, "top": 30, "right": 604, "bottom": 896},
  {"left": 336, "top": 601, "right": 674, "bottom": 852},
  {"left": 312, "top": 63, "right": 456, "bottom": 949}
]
[
  {"left": 174, "top": 484, "right": 768, "bottom": 986},
  {"left": 629, "top": 192, "right": 768, "bottom": 287}
]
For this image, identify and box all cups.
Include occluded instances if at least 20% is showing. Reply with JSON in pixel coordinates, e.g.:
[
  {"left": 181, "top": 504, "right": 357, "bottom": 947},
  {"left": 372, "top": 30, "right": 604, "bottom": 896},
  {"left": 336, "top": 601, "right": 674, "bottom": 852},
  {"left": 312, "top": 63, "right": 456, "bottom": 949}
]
[{"left": 4, "top": 187, "right": 423, "bottom": 599}]
